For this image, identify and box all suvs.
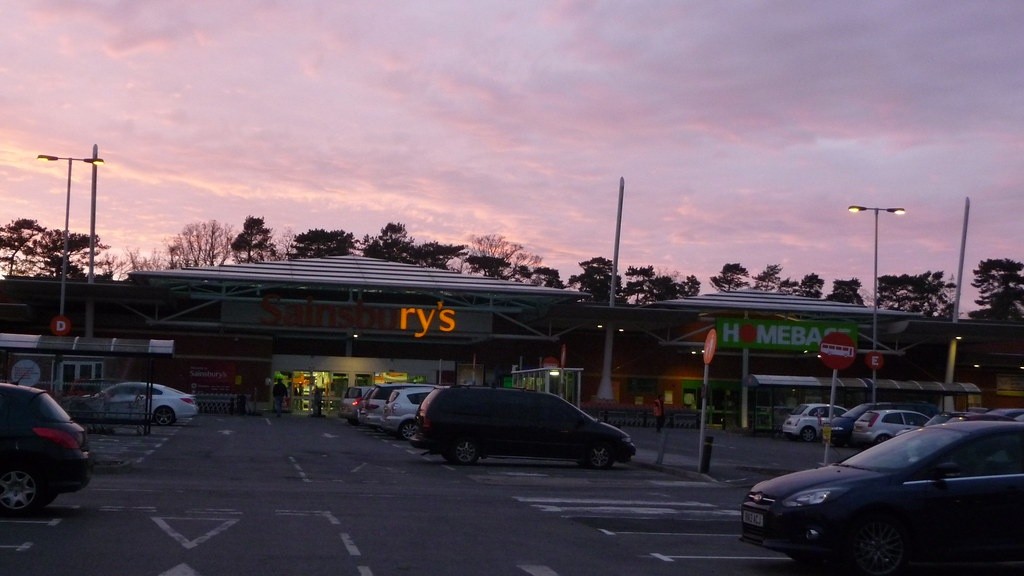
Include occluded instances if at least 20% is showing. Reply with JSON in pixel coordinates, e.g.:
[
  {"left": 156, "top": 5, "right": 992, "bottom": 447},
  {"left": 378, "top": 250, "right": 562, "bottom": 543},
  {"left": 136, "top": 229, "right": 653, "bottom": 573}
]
[
  {"left": 358, "top": 382, "right": 438, "bottom": 433},
  {"left": 338, "top": 386, "right": 375, "bottom": 426},
  {"left": 831, "top": 401, "right": 939, "bottom": 447},
  {"left": 408, "top": 382, "right": 637, "bottom": 470}
]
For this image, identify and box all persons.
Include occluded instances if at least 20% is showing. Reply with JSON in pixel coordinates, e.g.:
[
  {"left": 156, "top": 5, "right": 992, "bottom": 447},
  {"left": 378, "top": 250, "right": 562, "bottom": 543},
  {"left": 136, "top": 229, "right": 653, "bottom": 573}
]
[
  {"left": 653, "top": 393, "right": 665, "bottom": 433},
  {"left": 286, "top": 382, "right": 293, "bottom": 413},
  {"left": 272, "top": 379, "right": 288, "bottom": 417}
]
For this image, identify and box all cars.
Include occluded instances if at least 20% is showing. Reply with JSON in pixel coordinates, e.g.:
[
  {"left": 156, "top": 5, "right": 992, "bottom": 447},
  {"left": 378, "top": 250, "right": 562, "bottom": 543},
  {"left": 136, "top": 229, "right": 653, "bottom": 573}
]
[
  {"left": 782, "top": 403, "right": 849, "bottom": 442},
  {"left": 850, "top": 410, "right": 932, "bottom": 446},
  {"left": 71, "top": 382, "right": 200, "bottom": 427},
  {"left": 896, "top": 407, "right": 1024, "bottom": 443},
  {"left": 737, "top": 421, "right": 1023, "bottom": 576},
  {"left": 379, "top": 386, "right": 437, "bottom": 441},
  {"left": 0, "top": 382, "right": 94, "bottom": 519}
]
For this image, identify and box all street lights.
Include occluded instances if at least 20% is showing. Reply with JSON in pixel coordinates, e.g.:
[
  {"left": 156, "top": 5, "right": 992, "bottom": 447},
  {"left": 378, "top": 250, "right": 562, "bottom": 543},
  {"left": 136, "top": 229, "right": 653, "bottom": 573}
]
[
  {"left": 37, "top": 154, "right": 104, "bottom": 398},
  {"left": 847, "top": 205, "right": 906, "bottom": 405}
]
